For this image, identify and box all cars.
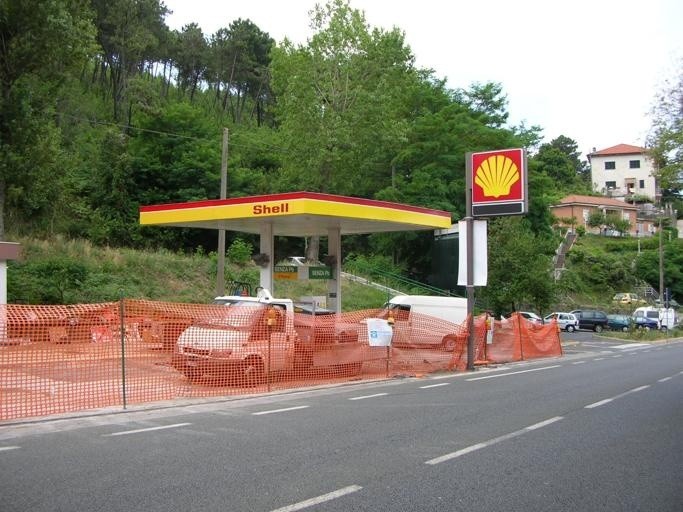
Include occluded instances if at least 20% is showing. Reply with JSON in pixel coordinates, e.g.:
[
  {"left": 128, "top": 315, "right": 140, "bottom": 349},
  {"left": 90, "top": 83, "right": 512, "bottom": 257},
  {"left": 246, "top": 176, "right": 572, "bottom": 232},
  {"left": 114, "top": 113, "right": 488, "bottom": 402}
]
[
  {"left": 612, "top": 293, "right": 647, "bottom": 305},
  {"left": 653, "top": 298, "right": 680, "bottom": 309},
  {"left": 500, "top": 308, "right": 659, "bottom": 335}
]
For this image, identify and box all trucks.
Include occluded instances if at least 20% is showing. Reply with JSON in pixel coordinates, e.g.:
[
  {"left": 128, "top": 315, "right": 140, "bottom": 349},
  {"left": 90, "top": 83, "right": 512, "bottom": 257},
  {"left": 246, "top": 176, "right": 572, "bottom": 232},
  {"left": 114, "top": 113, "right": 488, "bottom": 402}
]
[
  {"left": 358, "top": 295, "right": 469, "bottom": 353},
  {"left": 632, "top": 305, "right": 679, "bottom": 333},
  {"left": 172, "top": 286, "right": 393, "bottom": 382}
]
